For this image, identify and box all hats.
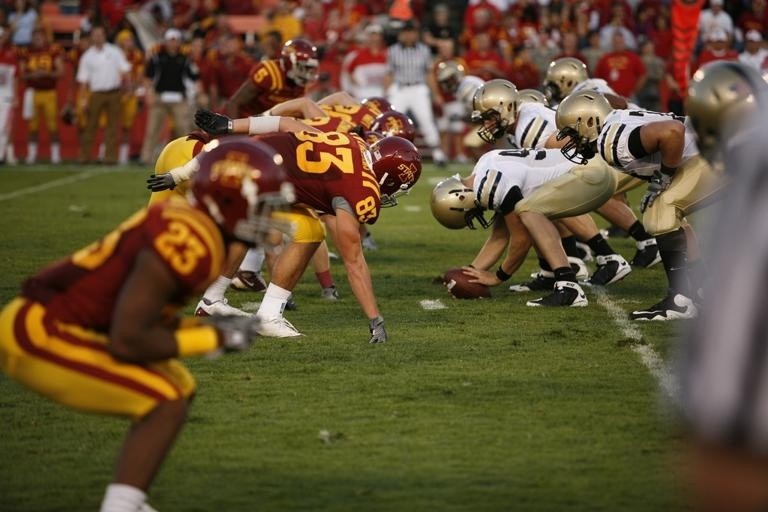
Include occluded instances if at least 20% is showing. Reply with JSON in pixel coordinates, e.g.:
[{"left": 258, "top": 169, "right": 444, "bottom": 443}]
[
  {"left": 399, "top": 19, "right": 418, "bottom": 32},
  {"left": 364, "top": 23, "right": 383, "bottom": 35},
  {"left": 164, "top": 27, "right": 182, "bottom": 40},
  {"left": 433, "top": 26, "right": 453, "bottom": 39}
]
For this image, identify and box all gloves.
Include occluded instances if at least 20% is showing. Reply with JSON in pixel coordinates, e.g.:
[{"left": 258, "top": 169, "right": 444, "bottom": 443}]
[
  {"left": 639, "top": 169, "right": 674, "bottom": 214},
  {"left": 365, "top": 315, "right": 389, "bottom": 346},
  {"left": 145, "top": 172, "right": 177, "bottom": 194},
  {"left": 192, "top": 106, "right": 234, "bottom": 135}
]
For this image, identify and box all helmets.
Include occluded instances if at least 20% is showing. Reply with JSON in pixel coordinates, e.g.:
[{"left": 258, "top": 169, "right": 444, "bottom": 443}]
[
  {"left": 543, "top": 56, "right": 590, "bottom": 105},
  {"left": 429, "top": 176, "right": 499, "bottom": 231},
  {"left": 435, "top": 60, "right": 465, "bottom": 96},
  {"left": 360, "top": 95, "right": 394, "bottom": 115},
  {"left": 470, "top": 78, "right": 520, "bottom": 145},
  {"left": 188, "top": 135, "right": 298, "bottom": 252},
  {"left": 361, "top": 129, "right": 384, "bottom": 145},
  {"left": 518, "top": 88, "right": 550, "bottom": 109},
  {"left": 685, "top": 57, "right": 767, "bottom": 177},
  {"left": 370, "top": 136, "right": 423, "bottom": 209},
  {"left": 553, "top": 88, "right": 614, "bottom": 166},
  {"left": 278, "top": 38, "right": 319, "bottom": 87},
  {"left": 370, "top": 111, "right": 416, "bottom": 143}
]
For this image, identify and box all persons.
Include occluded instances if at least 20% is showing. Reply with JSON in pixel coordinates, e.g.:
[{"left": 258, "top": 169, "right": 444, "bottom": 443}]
[
  {"left": 456, "top": 0, "right": 684, "bottom": 95},
  {"left": 149, "top": 139, "right": 253, "bottom": 322},
  {"left": 148, "top": 130, "right": 422, "bottom": 343},
  {"left": 155, "top": 109, "right": 384, "bottom": 299},
  {"left": 16, "top": 28, "right": 65, "bottom": 162},
  {"left": 318, "top": 91, "right": 391, "bottom": 116},
  {"left": 340, "top": 22, "right": 389, "bottom": 98},
  {"left": 431, "top": 149, "right": 619, "bottom": 309},
  {"left": 686, "top": 57, "right": 767, "bottom": 451},
  {"left": 1, "top": 141, "right": 286, "bottom": 512},
  {"left": 135, "top": 29, "right": 205, "bottom": 166},
  {"left": 2, "top": 3, "right": 339, "bottom": 60},
  {"left": 0, "top": 24, "right": 19, "bottom": 164},
  {"left": 555, "top": 90, "right": 730, "bottom": 321},
  {"left": 736, "top": 29, "right": 768, "bottom": 76},
  {"left": 76, "top": 27, "right": 130, "bottom": 164},
  {"left": 545, "top": 58, "right": 620, "bottom": 101},
  {"left": 472, "top": 79, "right": 663, "bottom": 284},
  {"left": 506, "top": 88, "right": 637, "bottom": 240},
  {"left": 690, "top": 25, "right": 738, "bottom": 80},
  {"left": 698, "top": 1, "right": 734, "bottom": 41},
  {"left": 387, "top": 27, "right": 448, "bottom": 167},
  {"left": 218, "top": 38, "right": 319, "bottom": 120},
  {"left": 254, "top": 98, "right": 416, "bottom": 255}
]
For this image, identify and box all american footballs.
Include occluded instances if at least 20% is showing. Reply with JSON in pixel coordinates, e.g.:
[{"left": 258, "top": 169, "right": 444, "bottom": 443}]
[{"left": 443, "top": 269, "right": 491, "bottom": 300}]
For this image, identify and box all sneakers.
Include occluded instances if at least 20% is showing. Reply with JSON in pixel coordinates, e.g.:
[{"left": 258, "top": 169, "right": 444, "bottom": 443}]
[
  {"left": 529, "top": 255, "right": 588, "bottom": 283},
  {"left": 254, "top": 314, "right": 302, "bottom": 339},
  {"left": 627, "top": 289, "right": 698, "bottom": 322},
  {"left": 578, "top": 253, "right": 633, "bottom": 289},
  {"left": 508, "top": 274, "right": 556, "bottom": 293},
  {"left": 229, "top": 270, "right": 268, "bottom": 293},
  {"left": 193, "top": 297, "right": 254, "bottom": 319},
  {"left": 526, "top": 281, "right": 590, "bottom": 309},
  {"left": 432, "top": 148, "right": 448, "bottom": 166},
  {"left": 628, "top": 237, "right": 663, "bottom": 270},
  {"left": 98, "top": 483, "right": 157, "bottom": 512}
]
